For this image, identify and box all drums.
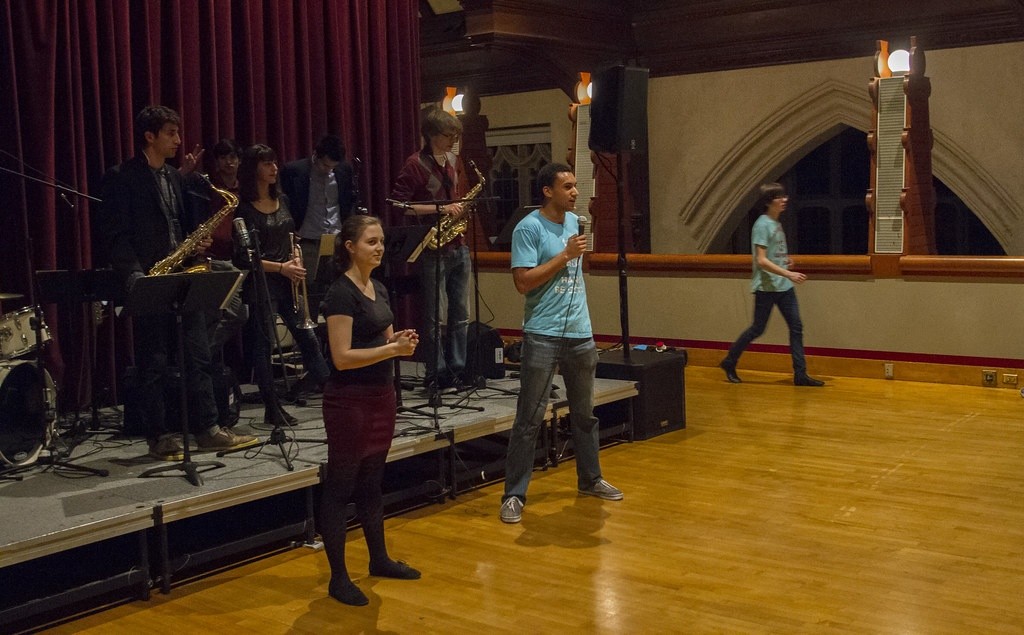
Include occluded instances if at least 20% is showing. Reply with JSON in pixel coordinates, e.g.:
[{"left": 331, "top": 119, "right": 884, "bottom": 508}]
[
  {"left": 1, "top": 360, "right": 60, "bottom": 473},
  {"left": 0, "top": 304, "right": 54, "bottom": 359}
]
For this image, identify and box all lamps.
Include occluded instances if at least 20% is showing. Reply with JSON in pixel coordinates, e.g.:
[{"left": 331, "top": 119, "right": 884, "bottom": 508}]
[
  {"left": 451, "top": 94, "right": 466, "bottom": 114},
  {"left": 888, "top": 48, "right": 910, "bottom": 77}
]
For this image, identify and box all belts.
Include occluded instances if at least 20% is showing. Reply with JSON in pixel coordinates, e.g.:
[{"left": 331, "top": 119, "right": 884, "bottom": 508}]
[{"left": 299, "top": 238, "right": 322, "bottom": 246}]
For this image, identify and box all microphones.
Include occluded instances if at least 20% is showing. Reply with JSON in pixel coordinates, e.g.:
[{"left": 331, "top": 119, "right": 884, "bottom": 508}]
[
  {"left": 232, "top": 218, "right": 251, "bottom": 247},
  {"left": 577, "top": 216, "right": 587, "bottom": 237},
  {"left": 385, "top": 198, "right": 414, "bottom": 210}
]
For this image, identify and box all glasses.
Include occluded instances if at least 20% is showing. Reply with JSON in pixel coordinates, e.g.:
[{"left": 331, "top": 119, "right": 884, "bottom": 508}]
[{"left": 440, "top": 131, "right": 458, "bottom": 138}]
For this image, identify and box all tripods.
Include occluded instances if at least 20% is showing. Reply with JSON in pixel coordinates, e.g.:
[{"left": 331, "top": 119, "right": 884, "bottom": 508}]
[
  {"left": 439, "top": 205, "right": 519, "bottom": 410},
  {"left": 217, "top": 224, "right": 328, "bottom": 471},
  {"left": 0, "top": 267, "right": 124, "bottom": 477},
  {"left": 396, "top": 202, "right": 485, "bottom": 429},
  {"left": 122, "top": 271, "right": 240, "bottom": 487}
]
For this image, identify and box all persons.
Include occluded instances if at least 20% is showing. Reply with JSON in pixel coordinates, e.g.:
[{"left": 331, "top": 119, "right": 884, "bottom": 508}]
[
  {"left": 500, "top": 161, "right": 625, "bottom": 524},
  {"left": 175, "top": 134, "right": 358, "bottom": 426},
  {"left": 89, "top": 105, "right": 259, "bottom": 461},
  {"left": 317, "top": 215, "right": 421, "bottom": 605},
  {"left": 389, "top": 109, "right": 473, "bottom": 390},
  {"left": 718, "top": 182, "right": 825, "bottom": 387}
]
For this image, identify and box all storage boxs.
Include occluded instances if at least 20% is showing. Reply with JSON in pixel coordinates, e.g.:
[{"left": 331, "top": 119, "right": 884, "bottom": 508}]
[{"left": 596, "top": 349, "right": 686, "bottom": 442}]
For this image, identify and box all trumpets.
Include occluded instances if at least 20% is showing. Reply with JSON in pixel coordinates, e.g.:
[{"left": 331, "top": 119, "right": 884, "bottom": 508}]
[{"left": 285, "top": 231, "right": 320, "bottom": 330}]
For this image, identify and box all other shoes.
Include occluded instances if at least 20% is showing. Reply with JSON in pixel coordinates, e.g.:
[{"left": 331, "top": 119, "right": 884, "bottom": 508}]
[
  {"left": 369, "top": 558, "right": 421, "bottom": 579},
  {"left": 794, "top": 370, "right": 825, "bottom": 385},
  {"left": 149, "top": 430, "right": 184, "bottom": 461},
  {"left": 329, "top": 578, "right": 369, "bottom": 605},
  {"left": 720, "top": 363, "right": 742, "bottom": 383},
  {"left": 197, "top": 426, "right": 259, "bottom": 452}
]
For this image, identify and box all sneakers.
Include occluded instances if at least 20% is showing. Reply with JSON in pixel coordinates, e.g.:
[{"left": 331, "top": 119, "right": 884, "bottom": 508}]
[
  {"left": 501, "top": 495, "right": 523, "bottom": 522},
  {"left": 578, "top": 478, "right": 623, "bottom": 500}
]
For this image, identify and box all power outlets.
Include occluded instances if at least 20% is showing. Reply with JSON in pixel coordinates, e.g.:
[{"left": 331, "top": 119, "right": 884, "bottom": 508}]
[
  {"left": 1003, "top": 373, "right": 1017, "bottom": 384},
  {"left": 885, "top": 363, "right": 894, "bottom": 378},
  {"left": 982, "top": 370, "right": 997, "bottom": 387}
]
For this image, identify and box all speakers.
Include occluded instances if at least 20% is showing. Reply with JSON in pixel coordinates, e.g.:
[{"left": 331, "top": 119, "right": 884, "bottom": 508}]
[
  {"left": 588, "top": 65, "right": 649, "bottom": 152},
  {"left": 464, "top": 321, "right": 505, "bottom": 380}
]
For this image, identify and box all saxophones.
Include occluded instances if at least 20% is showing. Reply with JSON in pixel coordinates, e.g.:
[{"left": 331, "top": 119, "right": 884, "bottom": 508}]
[
  {"left": 427, "top": 158, "right": 487, "bottom": 250},
  {"left": 149, "top": 170, "right": 239, "bottom": 278}
]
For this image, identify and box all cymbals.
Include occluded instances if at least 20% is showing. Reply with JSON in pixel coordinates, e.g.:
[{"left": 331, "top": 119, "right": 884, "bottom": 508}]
[{"left": 0, "top": 293, "right": 23, "bottom": 298}]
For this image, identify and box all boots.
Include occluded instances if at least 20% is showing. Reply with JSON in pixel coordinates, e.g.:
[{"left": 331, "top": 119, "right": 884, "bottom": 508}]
[{"left": 259, "top": 386, "right": 298, "bottom": 426}]
